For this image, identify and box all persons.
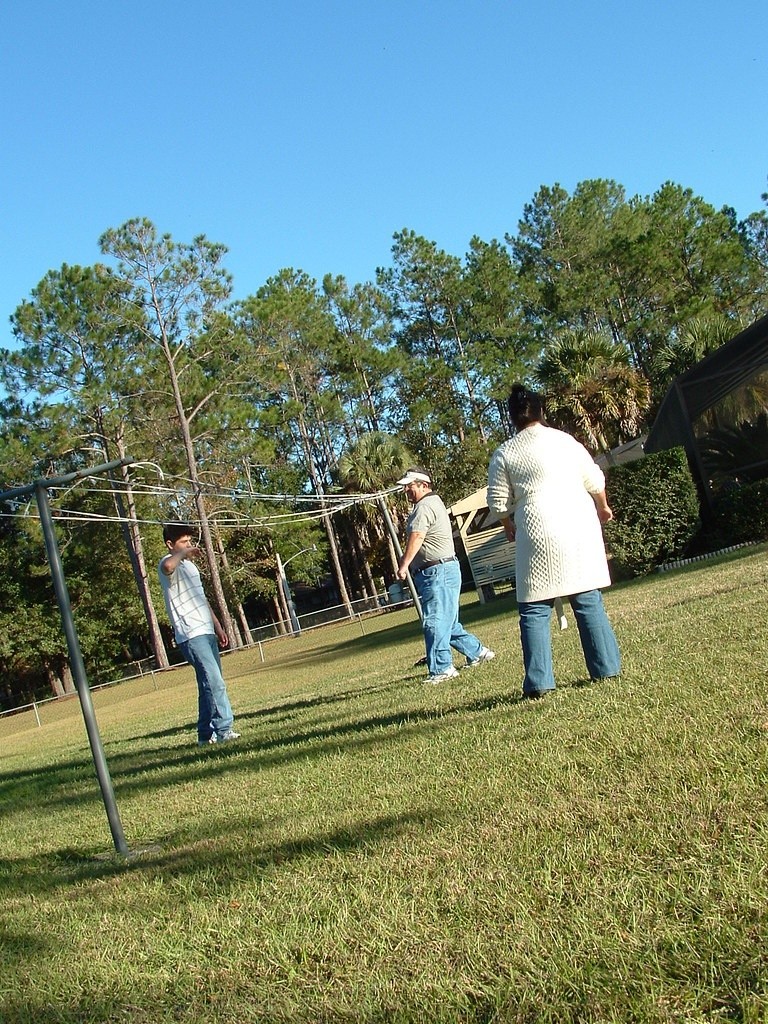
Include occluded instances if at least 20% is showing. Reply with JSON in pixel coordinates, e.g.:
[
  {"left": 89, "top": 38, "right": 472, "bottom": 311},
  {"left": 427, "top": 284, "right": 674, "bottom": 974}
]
[
  {"left": 487, "top": 379, "right": 621, "bottom": 697},
  {"left": 158, "top": 522, "right": 242, "bottom": 746},
  {"left": 397, "top": 469, "right": 495, "bottom": 684}
]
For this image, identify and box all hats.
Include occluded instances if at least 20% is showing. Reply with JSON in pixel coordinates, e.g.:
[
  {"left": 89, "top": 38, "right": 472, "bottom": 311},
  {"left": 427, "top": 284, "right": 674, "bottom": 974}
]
[{"left": 396, "top": 472, "right": 431, "bottom": 484}]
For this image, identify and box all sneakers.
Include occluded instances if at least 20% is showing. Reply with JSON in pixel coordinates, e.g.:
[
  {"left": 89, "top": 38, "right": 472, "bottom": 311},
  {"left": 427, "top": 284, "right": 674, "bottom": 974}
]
[
  {"left": 423, "top": 664, "right": 460, "bottom": 685},
  {"left": 216, "top": 730, "right": 240, "bottom": 742},
  {"left": 465, "top": 647, "right": 495, "bottom": 667},
  {"left": 198, "top": 735, "right": 218, "bottom": 746}
]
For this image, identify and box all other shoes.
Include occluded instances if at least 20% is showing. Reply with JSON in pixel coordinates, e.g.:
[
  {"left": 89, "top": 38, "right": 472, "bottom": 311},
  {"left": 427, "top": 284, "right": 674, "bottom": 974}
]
[{"left": 521, "top": 689, "right": 550, "bottom": 700}]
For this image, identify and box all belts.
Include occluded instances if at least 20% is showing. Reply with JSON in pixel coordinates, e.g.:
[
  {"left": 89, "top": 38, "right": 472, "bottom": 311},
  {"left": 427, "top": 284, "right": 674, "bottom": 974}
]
[{"left": 419, "top": 557, "right": 456, "bottom": 572}]
